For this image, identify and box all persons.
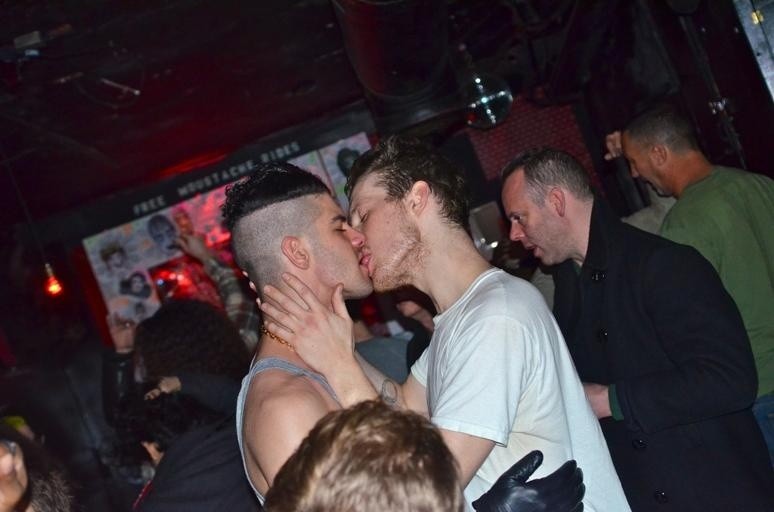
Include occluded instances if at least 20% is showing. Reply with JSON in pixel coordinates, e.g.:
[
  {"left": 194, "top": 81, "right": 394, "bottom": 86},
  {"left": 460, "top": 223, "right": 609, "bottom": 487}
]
[
  {"left": 242, "top": 135, "right": 632, "bottom": 511},
  {"left": 104, "top": 233, "right": 264, "bottom": 512},
  {"left": 222, "top": 162, "right": 586, "bottom": 512},
  {"left": 621, "top": 104, "right": 772, "bottom": 469},
  {"left": 503, "top": 147, "right": 774, "bottom": 512},
  {"left": 0, "top": 417, "right": 72, "bottom": 512},
  {"left": 345, "top": 284, "right": 437, "bottom": 385},
  {"left": 264, "top": 399, "right": 465, "bottom": 511}
]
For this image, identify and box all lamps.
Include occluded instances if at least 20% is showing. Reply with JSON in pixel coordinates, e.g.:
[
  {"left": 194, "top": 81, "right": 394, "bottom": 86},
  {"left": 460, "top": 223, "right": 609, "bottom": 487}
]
[{"left": 39, "top": 259, "right": 64, "bottom": 297}]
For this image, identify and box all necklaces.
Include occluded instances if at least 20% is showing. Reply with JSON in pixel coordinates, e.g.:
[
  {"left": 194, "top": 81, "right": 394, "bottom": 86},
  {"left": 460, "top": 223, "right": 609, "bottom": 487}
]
[{"left": 261, "top": 325, "right": 293, "bottom": 348}]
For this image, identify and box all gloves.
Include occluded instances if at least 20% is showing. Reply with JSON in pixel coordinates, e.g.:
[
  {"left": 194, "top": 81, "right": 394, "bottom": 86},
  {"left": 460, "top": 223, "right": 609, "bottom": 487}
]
[{"left": 472, "top": 450, "right": 585, "bottom": 512}]
